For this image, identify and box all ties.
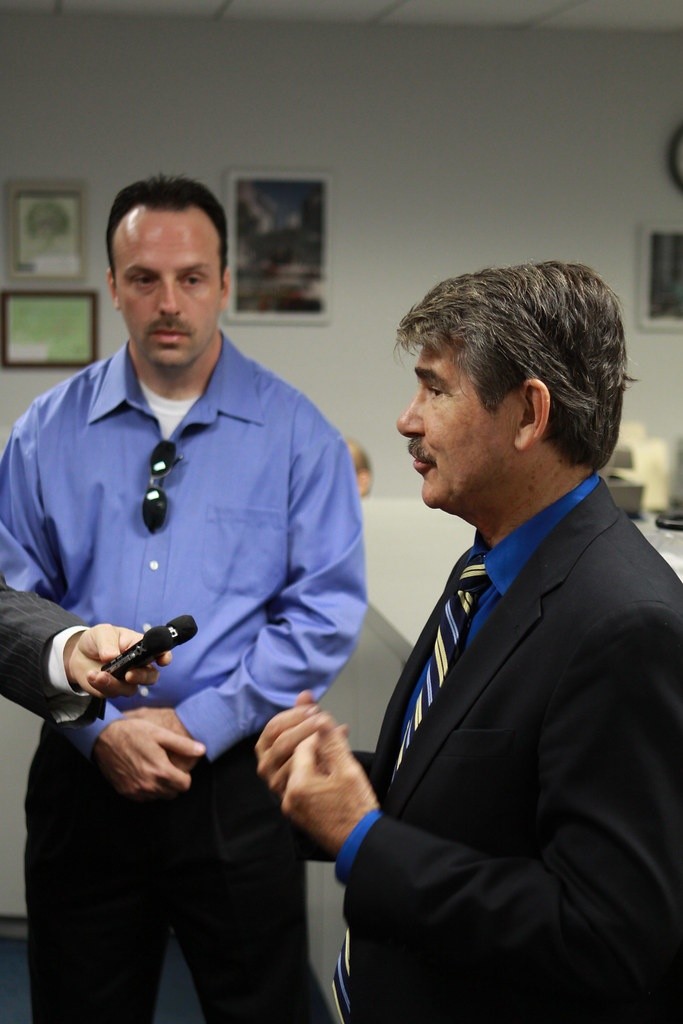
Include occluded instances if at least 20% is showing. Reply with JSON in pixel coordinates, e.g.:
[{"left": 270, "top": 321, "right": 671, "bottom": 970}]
[{"left": 328, "top": 553, "right": 492, "bottom": 1024}]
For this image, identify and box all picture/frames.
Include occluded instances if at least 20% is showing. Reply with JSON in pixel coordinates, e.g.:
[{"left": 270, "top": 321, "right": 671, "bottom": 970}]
[
  {"left": 637, "top": 223, "right": 683, "bottom": 332},
  {"left": 0, "top": 292, "right": 98, "bottom": 367},
  {"left": 10, "top": 177, "right": 87, "bottom": 281},
  {"left": 224, "top": 169, "right": 335, "bottom": 325}
]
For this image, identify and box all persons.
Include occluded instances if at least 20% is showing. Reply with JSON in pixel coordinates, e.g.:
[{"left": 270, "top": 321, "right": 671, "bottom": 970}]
[
  {"left": 255, "top": 260, "right": 683, "bottom": 1024},
  {"left": 0, "top": 175, "right": 366, "bottom": 1024}
]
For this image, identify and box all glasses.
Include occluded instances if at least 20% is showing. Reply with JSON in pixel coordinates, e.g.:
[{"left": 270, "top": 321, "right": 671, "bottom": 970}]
[{"left": 142, "top": 440, "right": 183, "bottom": 534}]
